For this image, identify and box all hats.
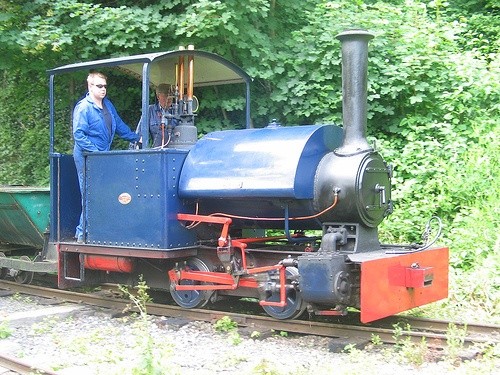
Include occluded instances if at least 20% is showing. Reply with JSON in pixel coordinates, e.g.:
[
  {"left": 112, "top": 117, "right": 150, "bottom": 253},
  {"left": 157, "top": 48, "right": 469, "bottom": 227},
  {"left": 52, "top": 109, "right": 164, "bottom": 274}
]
[{"left": 156, "top": 84, "right": 176, "bottom": 96}]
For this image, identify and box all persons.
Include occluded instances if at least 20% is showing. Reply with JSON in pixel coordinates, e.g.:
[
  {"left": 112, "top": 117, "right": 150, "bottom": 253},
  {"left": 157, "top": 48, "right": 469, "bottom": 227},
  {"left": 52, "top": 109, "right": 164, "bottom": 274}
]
[
  {"left": 71, "top": 70, "right": 143, "bottom": 245},
  {"left": 127, "top": 83, "right": 183, "bottom": 150}
]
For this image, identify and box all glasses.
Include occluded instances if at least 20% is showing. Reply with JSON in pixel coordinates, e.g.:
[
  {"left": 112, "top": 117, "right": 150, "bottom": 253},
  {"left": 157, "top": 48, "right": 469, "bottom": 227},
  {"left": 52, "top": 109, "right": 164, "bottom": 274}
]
[{"left": 91, "top": 83, "right": 107, "bottom": 88}]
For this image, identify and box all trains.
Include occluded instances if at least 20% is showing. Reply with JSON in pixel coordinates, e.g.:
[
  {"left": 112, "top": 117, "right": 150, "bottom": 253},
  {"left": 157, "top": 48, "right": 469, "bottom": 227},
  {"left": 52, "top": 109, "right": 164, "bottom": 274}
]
[{"left": 0, "top": 31, "right": 450, "bottom": 324}]
